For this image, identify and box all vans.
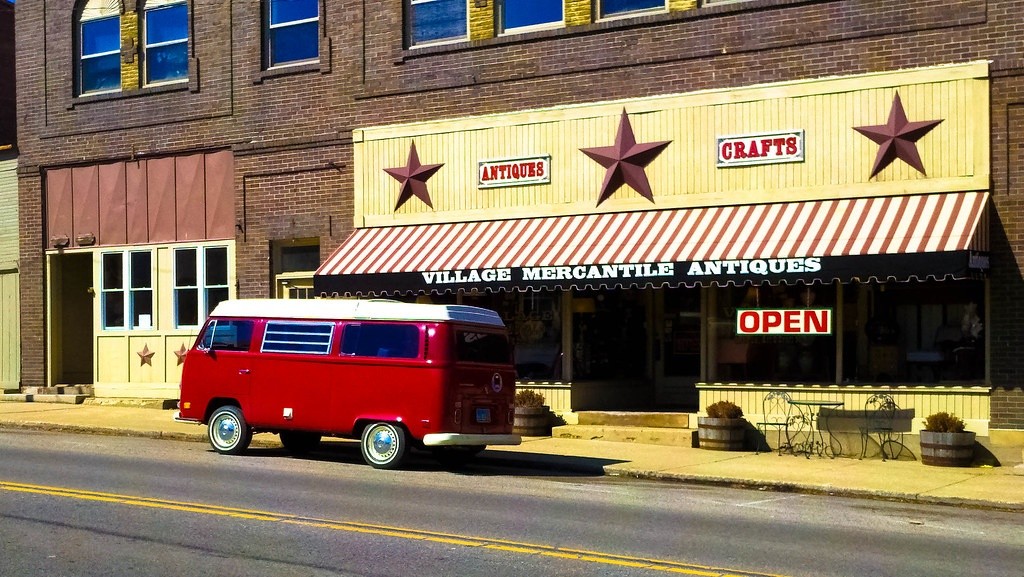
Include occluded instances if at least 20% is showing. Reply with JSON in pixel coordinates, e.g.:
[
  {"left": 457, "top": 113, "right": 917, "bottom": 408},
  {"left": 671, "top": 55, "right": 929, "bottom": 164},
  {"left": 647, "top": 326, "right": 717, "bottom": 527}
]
[{"left": 172, "top": 297, "right": 524, "bottom": 472}]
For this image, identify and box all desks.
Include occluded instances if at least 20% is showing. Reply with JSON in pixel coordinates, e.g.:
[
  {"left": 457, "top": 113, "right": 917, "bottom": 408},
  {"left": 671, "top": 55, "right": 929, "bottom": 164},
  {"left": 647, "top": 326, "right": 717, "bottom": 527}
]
[{"left": 788, "top": 399, "right": 844, "bottom": 458}]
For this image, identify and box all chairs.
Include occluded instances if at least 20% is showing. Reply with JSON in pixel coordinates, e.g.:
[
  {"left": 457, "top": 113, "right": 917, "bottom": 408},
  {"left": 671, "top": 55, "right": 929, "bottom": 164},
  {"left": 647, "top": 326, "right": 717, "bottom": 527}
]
[
  {"left": 857, "top": 393, "right": 896, "bottom": 459},
  {"left": 755, "top": 391, "right": 793, "bottom": 455}
]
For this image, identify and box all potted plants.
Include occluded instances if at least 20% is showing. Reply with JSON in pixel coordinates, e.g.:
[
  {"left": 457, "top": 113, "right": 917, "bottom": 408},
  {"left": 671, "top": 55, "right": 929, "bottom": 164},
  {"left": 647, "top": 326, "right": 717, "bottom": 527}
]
[
  {"left": 697, "top": 401, "right": 744, "bottom": 450},
  {"left": 512, "top": 386, "right": 550, "bottom": 437},
  {"left": 920, "top": 412, "right": 977, "bottom": 466}
]
